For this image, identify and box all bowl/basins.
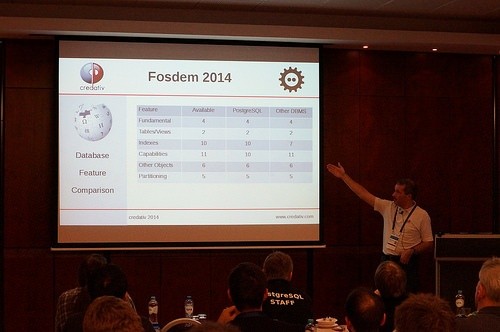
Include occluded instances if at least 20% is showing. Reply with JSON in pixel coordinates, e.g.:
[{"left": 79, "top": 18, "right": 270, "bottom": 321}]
[{"left": 316, "top": 318, "right": 337, "bottom": 324}]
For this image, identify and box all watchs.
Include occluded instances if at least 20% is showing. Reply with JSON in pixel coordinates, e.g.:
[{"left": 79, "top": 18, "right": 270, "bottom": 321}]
[{"left": 411, "top": 247, "right": 416, "bottom": 256}]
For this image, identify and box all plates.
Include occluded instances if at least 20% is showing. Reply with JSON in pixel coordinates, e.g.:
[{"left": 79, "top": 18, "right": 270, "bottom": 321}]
[{"left": 315, "top": 324, "right": 339, "bottom": 328}]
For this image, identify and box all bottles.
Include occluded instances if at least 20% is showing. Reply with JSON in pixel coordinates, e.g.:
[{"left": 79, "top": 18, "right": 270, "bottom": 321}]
[
  {"left": 149, "top": 297, "right": 158, "bottom": 325},
  {"left": 304, "top": 319, "right": 317, "bottom": 332},
  {"left": 184, "top": 296, "right": 194, "bottom": 327},
  {"left": 455, "top": 290, "right": 465, "bottom": 315}
]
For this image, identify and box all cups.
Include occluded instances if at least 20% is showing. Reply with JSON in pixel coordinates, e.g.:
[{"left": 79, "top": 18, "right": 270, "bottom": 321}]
[{"left": 462, "top": 307, "right": 471, "bottom": 314}]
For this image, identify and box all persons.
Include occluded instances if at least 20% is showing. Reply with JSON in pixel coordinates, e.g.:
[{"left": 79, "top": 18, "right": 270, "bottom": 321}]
[
  {"left": 54, "top": 251, "right": 500, "bottom": 332},
  {"left": 327, "top": 162, "right": 434, "bottom": 295}
]
